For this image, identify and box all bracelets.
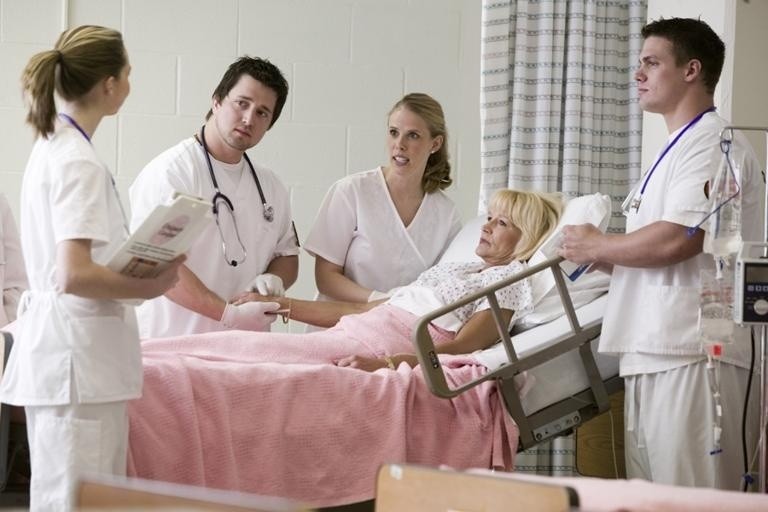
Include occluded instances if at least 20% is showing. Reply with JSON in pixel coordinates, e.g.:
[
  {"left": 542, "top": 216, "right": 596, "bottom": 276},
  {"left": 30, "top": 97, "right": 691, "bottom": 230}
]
[
  {"left": 384, "top": 358, "right": 396, "bottom": 371},
  {"left": 281, "top": 298, "right": 293, "bottom": 324}
]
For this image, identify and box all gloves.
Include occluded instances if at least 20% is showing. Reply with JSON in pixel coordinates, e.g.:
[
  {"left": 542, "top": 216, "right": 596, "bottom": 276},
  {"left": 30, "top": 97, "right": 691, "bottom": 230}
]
[
  {"left": 219, "top": 302, "right": 281, "bottom": 332},
  {"left": 245, "top": 272, "right": 284, "bottom": 297}
]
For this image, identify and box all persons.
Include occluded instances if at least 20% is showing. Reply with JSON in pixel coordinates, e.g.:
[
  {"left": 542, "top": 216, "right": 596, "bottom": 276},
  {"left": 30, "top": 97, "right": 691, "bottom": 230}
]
[
  {"left": 556, "top": 13, "right": 767, "bottom": 495},
  {"left": 1, "top": 191, "right": 34, "bottom": 336},
  {"left": 1, "top": 21, "right": 190, "bottom": 511},
  {"left": 300, "top": 87, "right": 468, "bottom": 337},
  {"left": 110, "top": 50, "right": 302, "bottom": 345},
  {"left": 220, "top": 183, "right": 567, "bottom": 374}
]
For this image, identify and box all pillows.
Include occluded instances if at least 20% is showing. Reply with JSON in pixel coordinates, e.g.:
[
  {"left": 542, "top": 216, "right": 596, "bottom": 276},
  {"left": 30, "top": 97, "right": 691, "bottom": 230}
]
[
  {"left": 433, "top": 192, "right": 612, "bottom": 324},
  {"left": 513, "top": 264, "right": 612, "bottom": 329}
]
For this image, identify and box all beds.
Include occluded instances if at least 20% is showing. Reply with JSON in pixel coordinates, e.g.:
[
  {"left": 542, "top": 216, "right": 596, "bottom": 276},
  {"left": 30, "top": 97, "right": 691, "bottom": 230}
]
[{"left": 0, "top": 248, "right": 625, "bottom": 508}]
